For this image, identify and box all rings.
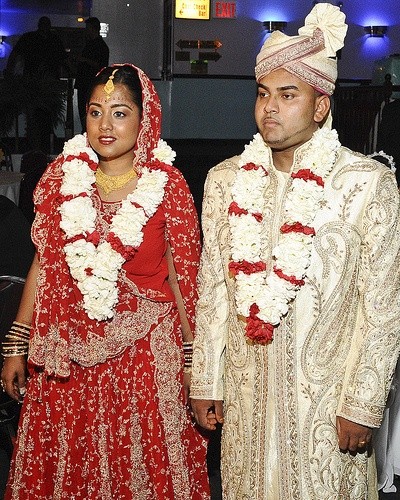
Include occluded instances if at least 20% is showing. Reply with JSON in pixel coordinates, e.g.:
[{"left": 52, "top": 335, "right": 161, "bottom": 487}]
[{"left": 359, "top": 441, "right": 366, "bottom": 445}]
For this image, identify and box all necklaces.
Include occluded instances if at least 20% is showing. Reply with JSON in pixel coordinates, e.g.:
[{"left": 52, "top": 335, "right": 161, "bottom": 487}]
[{"left": 95, "top": 166, "right": 137, "bottom": 201}]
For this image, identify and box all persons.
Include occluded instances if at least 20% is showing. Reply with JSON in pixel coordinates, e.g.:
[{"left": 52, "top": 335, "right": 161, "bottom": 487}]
[
  {"left": 1, "top": 64, "right": 210, "bottom": 500},
  {"left": 189, "top": 3, "right": 400, "bottom": 500},
  {"left": 30, "top": 17, "right": 110, "bottom": 134}
]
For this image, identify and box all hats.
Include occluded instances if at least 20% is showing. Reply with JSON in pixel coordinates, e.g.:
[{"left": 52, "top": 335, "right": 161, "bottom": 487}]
[{"left": 253, "top": 2, "right": 349, "bottom": 99}]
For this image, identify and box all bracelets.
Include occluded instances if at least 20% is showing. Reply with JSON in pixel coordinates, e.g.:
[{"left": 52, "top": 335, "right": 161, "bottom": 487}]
[
  {"left": 1, "top": 320, "right": 31, "bottom": 357},
  {"left": 182, "top": 341, "right": 194, "bottom": 374}
]
